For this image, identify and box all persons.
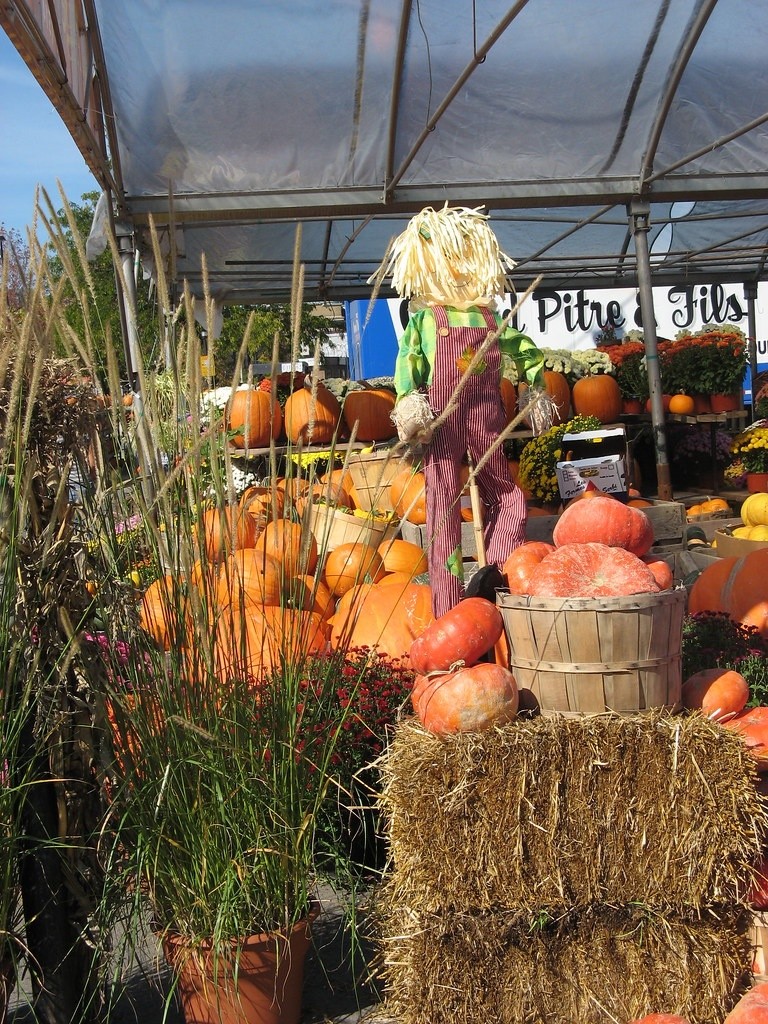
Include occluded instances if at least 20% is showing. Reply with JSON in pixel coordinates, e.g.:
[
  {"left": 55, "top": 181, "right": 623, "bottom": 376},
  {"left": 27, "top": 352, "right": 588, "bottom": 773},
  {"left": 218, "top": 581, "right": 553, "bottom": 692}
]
[{"left": 366, "top": 200, "right": 562, "bottom": 618}]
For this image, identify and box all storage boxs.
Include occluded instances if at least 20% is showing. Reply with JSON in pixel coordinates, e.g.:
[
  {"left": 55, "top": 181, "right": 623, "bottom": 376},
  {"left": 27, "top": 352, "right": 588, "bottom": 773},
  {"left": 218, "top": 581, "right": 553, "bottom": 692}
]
[{"left": 497, "top": 580, "right": 691, "bottom": 723}]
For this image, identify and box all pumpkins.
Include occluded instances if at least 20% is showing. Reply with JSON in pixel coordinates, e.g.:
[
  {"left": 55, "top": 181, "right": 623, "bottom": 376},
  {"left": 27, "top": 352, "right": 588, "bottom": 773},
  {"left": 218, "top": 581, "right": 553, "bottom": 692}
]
[{"left": 101, "top": 368, "right": 768, "bottom": 909}]
